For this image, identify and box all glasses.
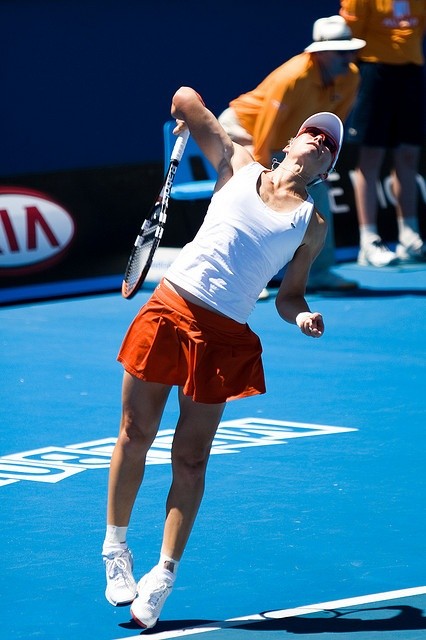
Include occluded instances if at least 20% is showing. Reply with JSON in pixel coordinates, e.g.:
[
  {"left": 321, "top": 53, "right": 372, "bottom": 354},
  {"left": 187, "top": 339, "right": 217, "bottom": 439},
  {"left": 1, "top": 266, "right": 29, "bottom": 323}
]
[{"left": 296, "top": 126, "right": 338, "bottom": 170}]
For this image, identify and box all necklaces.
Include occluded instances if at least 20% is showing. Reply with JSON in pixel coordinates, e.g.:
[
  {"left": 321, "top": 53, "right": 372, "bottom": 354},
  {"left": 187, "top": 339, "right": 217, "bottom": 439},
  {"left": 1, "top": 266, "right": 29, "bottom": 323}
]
[{"left": 271, "top": 157, "right": 309, "bottom": 186}]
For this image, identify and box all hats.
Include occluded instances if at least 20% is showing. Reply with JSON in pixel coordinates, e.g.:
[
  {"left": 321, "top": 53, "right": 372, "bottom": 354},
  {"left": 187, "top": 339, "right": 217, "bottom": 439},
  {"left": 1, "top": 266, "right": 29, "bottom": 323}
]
[
  {"left": 304, "top": 15, "right": 367, "bottom": 53},
  {"left": 298, "top": 112, "right": 344, "bottom": 173}
]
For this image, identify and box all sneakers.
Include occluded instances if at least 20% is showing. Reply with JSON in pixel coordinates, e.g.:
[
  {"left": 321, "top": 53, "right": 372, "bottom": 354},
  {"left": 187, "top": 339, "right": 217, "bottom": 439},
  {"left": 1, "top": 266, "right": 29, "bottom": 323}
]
[
  {"left": 129, "top": 569, "right": 173, "bottom": 628},
  {"left": 396, "top": 237, "right": 426, "bottom": 262},
  {"left": 358, "top": 237, "right": 401, "bottom": 268},
  {"left": 306, "top": 269, "right": 359, "bottom": 294},
  {"left": 102, "top": 549, "right": 137, "bottom": 606}
]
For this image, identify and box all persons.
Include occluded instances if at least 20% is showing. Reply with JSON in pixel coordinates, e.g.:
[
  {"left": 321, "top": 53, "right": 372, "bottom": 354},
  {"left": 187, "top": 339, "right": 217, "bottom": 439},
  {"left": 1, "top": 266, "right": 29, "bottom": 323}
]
[
  {"left": 217, "top": 16, "right": 367, "bottom": 301},
  {"left": 102, "top": 86, "right": 344, "bottom": 627},
  {"left": 340, "top": 0, "right": 425, "bottom": 267}
]
